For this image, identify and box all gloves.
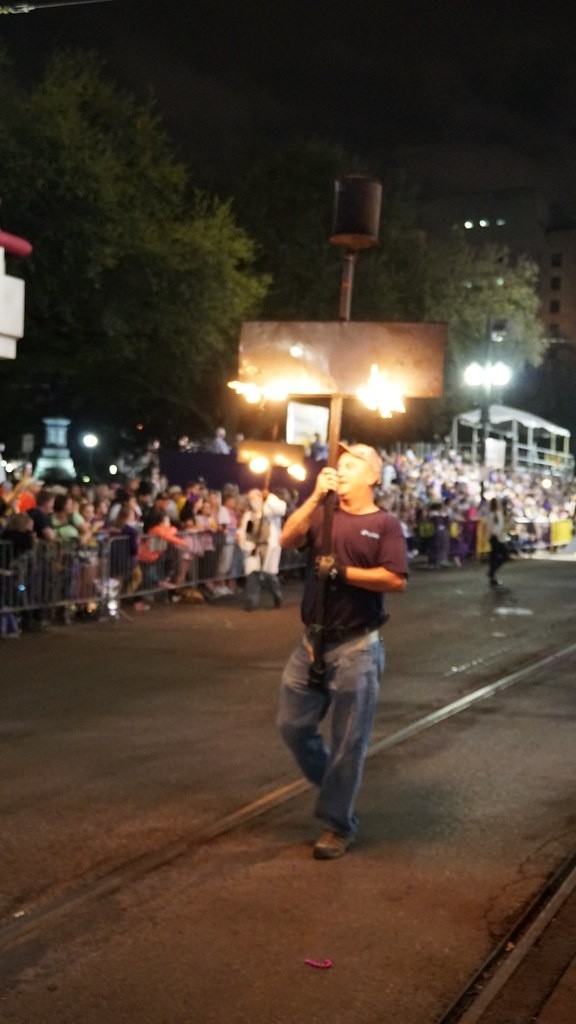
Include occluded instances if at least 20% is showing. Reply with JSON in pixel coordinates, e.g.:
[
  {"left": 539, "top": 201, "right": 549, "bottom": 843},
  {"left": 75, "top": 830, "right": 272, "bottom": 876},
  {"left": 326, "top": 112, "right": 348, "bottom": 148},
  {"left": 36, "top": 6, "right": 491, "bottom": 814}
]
[{"left": 315, "top": 553, "right": 346, "bottom": 589}]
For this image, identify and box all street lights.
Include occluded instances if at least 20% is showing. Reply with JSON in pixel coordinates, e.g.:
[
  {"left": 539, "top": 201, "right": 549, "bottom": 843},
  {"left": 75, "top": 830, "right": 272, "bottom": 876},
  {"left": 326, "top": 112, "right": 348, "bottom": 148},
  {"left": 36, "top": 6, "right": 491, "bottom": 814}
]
[{"left": 460, "top": 294, "right": 515, "bottom": 468}]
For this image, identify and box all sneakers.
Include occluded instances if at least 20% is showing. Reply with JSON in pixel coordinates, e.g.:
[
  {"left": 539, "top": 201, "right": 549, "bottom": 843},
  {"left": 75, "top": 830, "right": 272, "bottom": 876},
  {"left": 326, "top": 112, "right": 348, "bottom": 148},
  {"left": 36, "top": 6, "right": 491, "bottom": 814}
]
[{"left": 313, "top": 830, "right": 357, "bottom": 859}]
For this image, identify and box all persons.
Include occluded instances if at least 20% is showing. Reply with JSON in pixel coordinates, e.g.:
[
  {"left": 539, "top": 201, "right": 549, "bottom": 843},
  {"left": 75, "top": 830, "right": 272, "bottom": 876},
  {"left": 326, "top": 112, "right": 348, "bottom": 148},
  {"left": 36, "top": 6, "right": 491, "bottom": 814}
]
[
  {"left": 274, "top": 444, "right": 408, "bottom": 858},
  {"left": 0, "top": 426, "right": 576, "bottom": 639}
]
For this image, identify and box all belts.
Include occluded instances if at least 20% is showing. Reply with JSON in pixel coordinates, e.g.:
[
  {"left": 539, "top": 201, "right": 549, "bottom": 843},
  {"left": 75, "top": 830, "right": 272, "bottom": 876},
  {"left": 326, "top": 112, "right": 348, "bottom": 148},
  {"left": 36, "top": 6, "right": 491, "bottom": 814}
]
[{"left": 308, "top": 610, "right": 391, "bottom": 644}]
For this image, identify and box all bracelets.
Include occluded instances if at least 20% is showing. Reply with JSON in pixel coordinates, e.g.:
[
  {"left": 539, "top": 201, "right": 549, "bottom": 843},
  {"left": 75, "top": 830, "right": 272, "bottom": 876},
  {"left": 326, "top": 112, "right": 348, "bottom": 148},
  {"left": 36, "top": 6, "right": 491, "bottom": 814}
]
[{"left": 329, "top": 563, "right": 345, "bottom": 584}]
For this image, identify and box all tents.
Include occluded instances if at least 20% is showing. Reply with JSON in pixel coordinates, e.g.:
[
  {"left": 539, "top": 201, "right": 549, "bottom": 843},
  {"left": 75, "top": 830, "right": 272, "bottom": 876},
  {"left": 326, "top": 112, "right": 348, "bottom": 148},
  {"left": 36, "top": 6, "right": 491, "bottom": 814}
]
[{"left": 452, "top": 404, "right": 571, "bottom": 472}]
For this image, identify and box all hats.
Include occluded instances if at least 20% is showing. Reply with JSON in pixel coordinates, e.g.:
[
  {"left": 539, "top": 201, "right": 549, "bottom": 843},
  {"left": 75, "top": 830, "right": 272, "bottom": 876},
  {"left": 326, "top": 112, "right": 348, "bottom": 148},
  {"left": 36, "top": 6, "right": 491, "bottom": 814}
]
[{"left": 339, "top": 442, "right": 383, "bottom": 486}]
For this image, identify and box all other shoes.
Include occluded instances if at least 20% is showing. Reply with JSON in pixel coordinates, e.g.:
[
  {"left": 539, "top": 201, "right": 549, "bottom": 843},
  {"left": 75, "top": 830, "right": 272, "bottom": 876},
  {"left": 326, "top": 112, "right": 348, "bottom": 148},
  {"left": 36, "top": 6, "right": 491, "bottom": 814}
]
[{"left": 19, "top": 586, "right": 285, "bottom": 634}]
